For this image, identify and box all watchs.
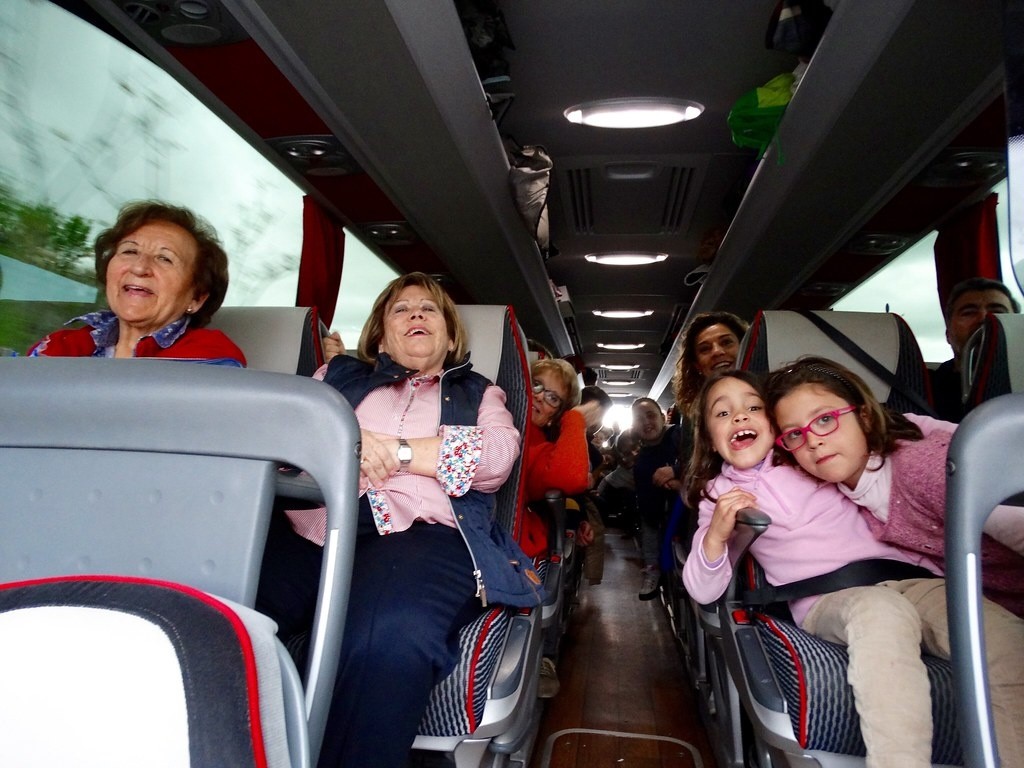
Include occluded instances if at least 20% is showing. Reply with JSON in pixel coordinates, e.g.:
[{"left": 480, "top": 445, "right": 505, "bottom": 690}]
[{"left": 397, "top": 438, "right": 413, "bottom": 472}]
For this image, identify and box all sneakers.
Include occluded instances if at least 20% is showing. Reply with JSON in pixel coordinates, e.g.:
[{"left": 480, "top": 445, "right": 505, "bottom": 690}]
[
  {"left": 536, "top": 656, "right": 560, "bottom": 698},
  {"left": 638, "top": 568, "right": 661, "bottom": 601}
]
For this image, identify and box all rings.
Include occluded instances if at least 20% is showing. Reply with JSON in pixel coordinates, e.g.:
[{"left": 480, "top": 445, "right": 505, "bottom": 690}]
[{"left": 360, "top": 459, "right": 364, "bottom": 464}]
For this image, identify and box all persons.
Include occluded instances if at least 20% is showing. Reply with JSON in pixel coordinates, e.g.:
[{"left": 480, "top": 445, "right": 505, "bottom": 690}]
[
  {"left": 580, "top": 385, "right": 681, "bottom": 600},
  {"left": 514, "top": 358, "right": 590, "bottom": 698},
  {"left": 675, "top": 311, "right": 751, "bottom": 416},
  {"left": 25, "top": 200, "right": 246, "bottom": 368},
  {"left": 920, "top": 276, "right": 1019, "bottom": 413},
  {"left": 683, "top": 356, "right": 1024, "bottom": 768},
  {"left": 256, "top": 271, "right": 519, "bottom": 767}
]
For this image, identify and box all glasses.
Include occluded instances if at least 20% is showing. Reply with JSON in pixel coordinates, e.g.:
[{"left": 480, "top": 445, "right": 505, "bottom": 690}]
[
  {"left": 776, "top": 406, "right": 856, "bottom": 452},
  {"left": 531, "top": 376, "right": 565, "bottom": 409}
]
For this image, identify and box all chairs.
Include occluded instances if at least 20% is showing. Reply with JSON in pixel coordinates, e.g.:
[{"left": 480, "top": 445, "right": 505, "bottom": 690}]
[
  {"left": 0, "top": 304, "right": 545, "bottom": 768},
  {"left": 672, "top": 308, "right": 1024, "bottom": 768}
]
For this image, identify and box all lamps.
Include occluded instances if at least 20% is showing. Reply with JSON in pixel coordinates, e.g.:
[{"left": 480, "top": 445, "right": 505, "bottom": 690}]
[
  {"left": 561, "top": 95, "right": 710, "bottom": 130},
  {"left": 583, "top": 249, "right": 670, "bottom": 266},
  {"left": 591, "top": 308, "right": 655, "bottom": 319},
  {"left": 600, "top": 362, "right": 641, "bottom": 372},
  {"left": 596, "top": 341, "right": 646, "bottom": 351}
]
